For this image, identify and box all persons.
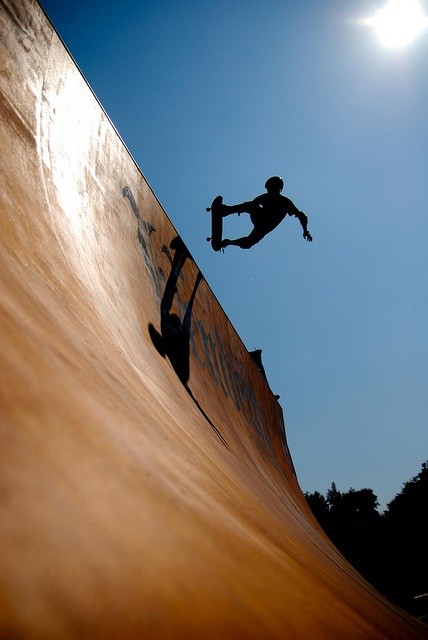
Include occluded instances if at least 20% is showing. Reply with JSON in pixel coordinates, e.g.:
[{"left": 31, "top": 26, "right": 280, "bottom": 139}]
[{"left": 219, "top": 175, "right": 313, "bottom": 254}]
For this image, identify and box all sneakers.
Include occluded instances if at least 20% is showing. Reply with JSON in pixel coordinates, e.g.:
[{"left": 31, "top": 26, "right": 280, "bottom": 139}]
[
  {"left": 223, "top": 204, "right": 230, "bottom": 217},
  {"left": 222, "top": 239, "right": 230, "bottom": 248}
]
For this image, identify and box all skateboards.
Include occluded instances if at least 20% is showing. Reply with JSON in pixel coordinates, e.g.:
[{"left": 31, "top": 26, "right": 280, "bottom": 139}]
[{"left": 206, "top": 195, "right": 223, "bottom": 251}]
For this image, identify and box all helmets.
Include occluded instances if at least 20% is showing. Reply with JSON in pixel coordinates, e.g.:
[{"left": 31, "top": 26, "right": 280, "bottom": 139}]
[{"left": 264, "top": 176, "right": 284, "bottom": 192}]
